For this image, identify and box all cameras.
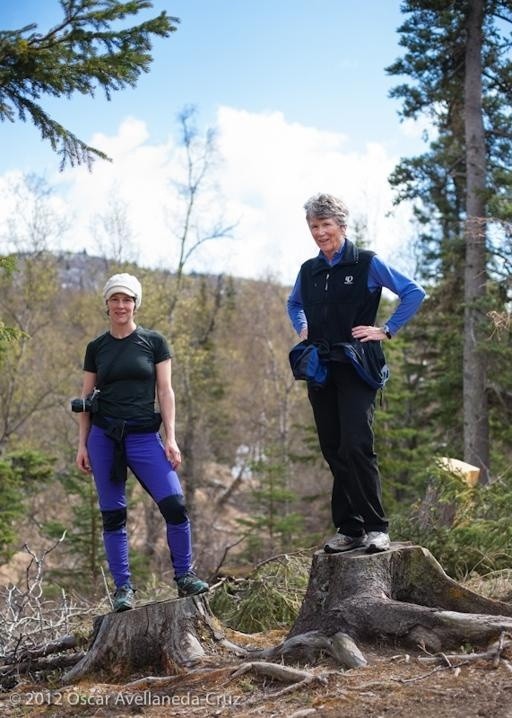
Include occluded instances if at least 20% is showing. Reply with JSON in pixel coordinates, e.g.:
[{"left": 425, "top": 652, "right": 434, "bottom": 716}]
[{"left": 71, "top": 395, "right": 99, "bottom": 415}]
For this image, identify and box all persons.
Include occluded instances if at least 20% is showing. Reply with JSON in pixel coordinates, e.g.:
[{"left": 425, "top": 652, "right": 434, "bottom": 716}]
[
  {"left": 75, "top": 272, "right": 211, "bottom": 610},
  {"left": 287, "top": 194, "right": 427, "bottom": 552}
]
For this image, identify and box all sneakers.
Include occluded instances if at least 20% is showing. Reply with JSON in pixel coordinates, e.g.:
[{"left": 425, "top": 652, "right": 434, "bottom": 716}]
[
  {"left": 113, "top": 584, "right": 136, "bottom": 612},
  {"left": 176, "top": 573, "right": 209, "bottom": 598},
  {"left": 324, "top": 531, "right": 391, "bottom": 553}
]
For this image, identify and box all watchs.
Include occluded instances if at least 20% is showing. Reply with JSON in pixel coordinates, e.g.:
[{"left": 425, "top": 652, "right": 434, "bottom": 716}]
[{"left": 382, "top": 326, "right": 391, "bottom": 339}]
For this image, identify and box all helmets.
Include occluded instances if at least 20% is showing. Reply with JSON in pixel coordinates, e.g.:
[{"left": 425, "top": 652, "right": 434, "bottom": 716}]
[{"left": 102, "top": 273, "right": 142, "bottom": 312}]
